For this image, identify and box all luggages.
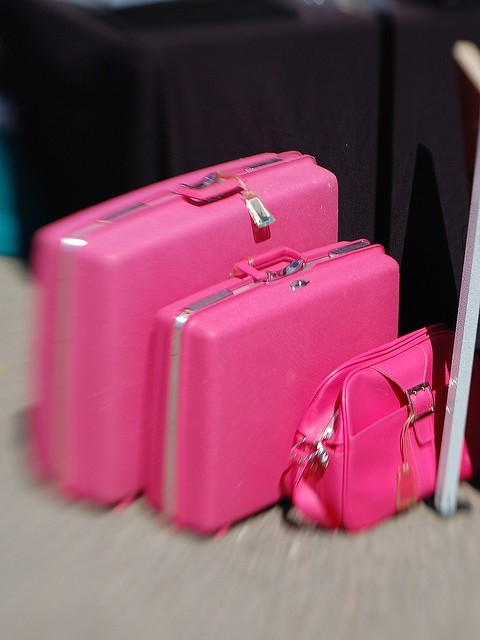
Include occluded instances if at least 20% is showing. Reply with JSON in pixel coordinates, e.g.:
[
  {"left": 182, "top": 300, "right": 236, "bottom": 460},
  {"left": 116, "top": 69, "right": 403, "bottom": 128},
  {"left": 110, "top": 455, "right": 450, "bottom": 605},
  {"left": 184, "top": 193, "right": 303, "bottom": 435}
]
[
  {"left": 26, "top": 150, "right": 338, "bottom": 515},
  {"left": 144, "top": 239, "right": 399, "bottom": 535}
]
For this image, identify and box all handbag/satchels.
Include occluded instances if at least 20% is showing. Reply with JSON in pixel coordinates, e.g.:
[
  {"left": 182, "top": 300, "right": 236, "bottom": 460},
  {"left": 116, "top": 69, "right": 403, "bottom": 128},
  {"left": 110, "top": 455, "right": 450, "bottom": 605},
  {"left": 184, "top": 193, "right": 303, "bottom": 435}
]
[{"left": 278, "top": 321, "right": 480, "bottom": 531}]
